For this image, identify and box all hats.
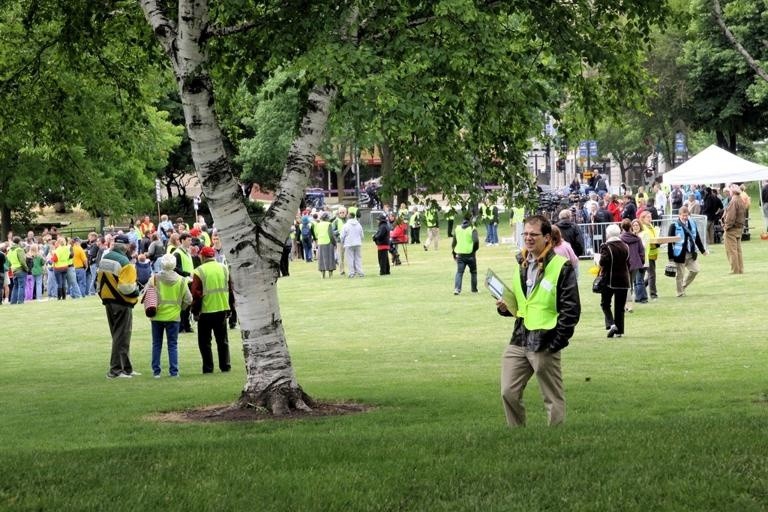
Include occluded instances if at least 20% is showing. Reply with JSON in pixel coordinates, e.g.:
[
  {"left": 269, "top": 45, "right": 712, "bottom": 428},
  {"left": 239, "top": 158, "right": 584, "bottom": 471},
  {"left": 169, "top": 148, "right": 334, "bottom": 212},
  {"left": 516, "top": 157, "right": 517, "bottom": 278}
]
[
  {"left": 302, "top": 217, "right": 308, "bottom": 222},
  {"left": 200, "top": 248, "right": 215, "bottom": 257},
  {"left": 115, "top": 235, "right": 129, "bottom": 244},
  {"left": 376, "top": 214, "right": 388, "bottom": 223}
]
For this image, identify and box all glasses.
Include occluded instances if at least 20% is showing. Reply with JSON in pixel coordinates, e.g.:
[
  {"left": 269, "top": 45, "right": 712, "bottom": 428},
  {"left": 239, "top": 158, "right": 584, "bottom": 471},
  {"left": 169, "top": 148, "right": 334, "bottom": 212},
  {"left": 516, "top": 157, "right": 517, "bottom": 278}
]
[
  {"left": 340, "top": 212, "right": 345, "bottom": 214},
  {"left": 521, "top": 232, "right": 546, "bottom": 238}
]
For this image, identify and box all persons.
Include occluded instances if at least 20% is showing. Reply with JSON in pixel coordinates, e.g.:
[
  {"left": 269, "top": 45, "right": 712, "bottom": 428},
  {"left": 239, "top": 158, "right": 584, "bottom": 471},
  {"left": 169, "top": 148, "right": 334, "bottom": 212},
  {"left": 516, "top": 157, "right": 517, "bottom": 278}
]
[
  {"left": 65, "top": 231, "right": 116, "bottom": 299},
  {"left": 277, "top": 232, "right": 292, "bottom": 277},
  {"left": 423, "top": 199, "right": 440, "bottom": 252},
  {"left": 126, "top": 213, "right": 225, "bottom": 268},
  {"left": 24, "top": 226, "right": 57, "bottom": 302},
  {"left": 0, "top": 241, "right": 8, "bottom": 304},
  {"left": 410, "top": 206, "right": 421, "bottom": 244},
  {"left": 51, "top": 237, "right": 71, "bottom": 300},
  {"left": 493, "top": 214, "right": 583, "bottom": 429},
  {"left": 528, "top": 167, "right": 719, "bottom": 231},
  {"left": 91, "top": 233, "right": 143, "bottom": 380},
  {"left": 340, "top": 212, "right": 365, "bottom": 277},
  {"left": 549, "top": 225, "right": 580, "bottom": 277},
  {"left": 445, "top": 201, "right": 456, "bottom": 237},
  {"left": 450, "top": 211, "right": 480, "bottom": 295},
  {"left": 384, "top": 213, "right": 402, "bottom": 266},
  {"left": 332, "top": 206, "right": 351, "bottom": 276},
  {"left": 618, "top": 217, "right": 649, "bottom": 304},
  {"left": 227, "top": 274, "right": 237, "bottom": 328},
  {"left": 635, "top": 211, "right": 664, "bottom": 300},
  {"left": 138, "top": 251, "right": 194, "bottom": 379},
  {"left": 365, "top": 182, "right": 382, "bottom": 208},
  {"left": 758, "top": 178, "right": 768, "bottom": 240},
  {"left": 667, "top": 206, "right": 709, "bottom": 297},
  {"left": 485, "top": 196, "right": 499, "bottom": 245},
  {"left": 397, "top": 202, "right": 410, "bottom": 245},
  {"left": 188, "top": 245, "right": 237, "bottom": 374},
  {"left": 6, "top": 237, "right": 30, "bottom": 305},
  {"left": 172, "top": 233, "right": 196, "bottom": 335},
  {"left": 720, "top": 183, "right": 746, "bottom": 276},
  {"left": 593, "top": 223, "right": 630, "bottom": 338},
  {"left": 289, "top": 206, "right": 318, "bottom": 263},
  {"left": 370, "top": 212, "right": 391, "bottom": 275},
  {"left": 312, "top": 212, "right": 338, "bottom": 280},
  {"left": 478, "top": 198, "right": 490, "bottom": 242},
  {"left": 629, "top": 218, "right": 650, "bottom": 302}
]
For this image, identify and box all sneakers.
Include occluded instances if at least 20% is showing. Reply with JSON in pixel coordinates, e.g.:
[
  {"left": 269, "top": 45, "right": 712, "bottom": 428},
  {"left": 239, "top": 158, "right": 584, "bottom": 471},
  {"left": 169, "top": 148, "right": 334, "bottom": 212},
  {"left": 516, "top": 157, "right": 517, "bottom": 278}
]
[
  {"left": 608, "top": 326, "right": 618, "bottom": 337},
  {"left": 454, "top": 290, "right": 459, "bottom": 294},
  {"left": 106, "top": 373, "right": 132, "bottom": 380},
  {"left": 617, "top": 334, "right": 624, "bottom": 337},
  {"left": 123, "top": 370, "right": 142, "bottom": 376}
]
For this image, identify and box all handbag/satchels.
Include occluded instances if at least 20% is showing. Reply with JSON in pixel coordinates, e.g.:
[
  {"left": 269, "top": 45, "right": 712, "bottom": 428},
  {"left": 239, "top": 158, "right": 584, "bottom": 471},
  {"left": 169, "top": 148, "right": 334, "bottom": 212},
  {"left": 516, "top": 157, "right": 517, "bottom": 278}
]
[
  {"left": 693, "top": 252, "right": 697, "bottom": 260},
  {"left": 593, "top": 267, "right": 609, "bottom": 293},
  {"left": 307, "top": 238, "right": 312, "bottom": 248},
  {"left": 140, "top": 280, "right": 158, "bottom": 317}
]
